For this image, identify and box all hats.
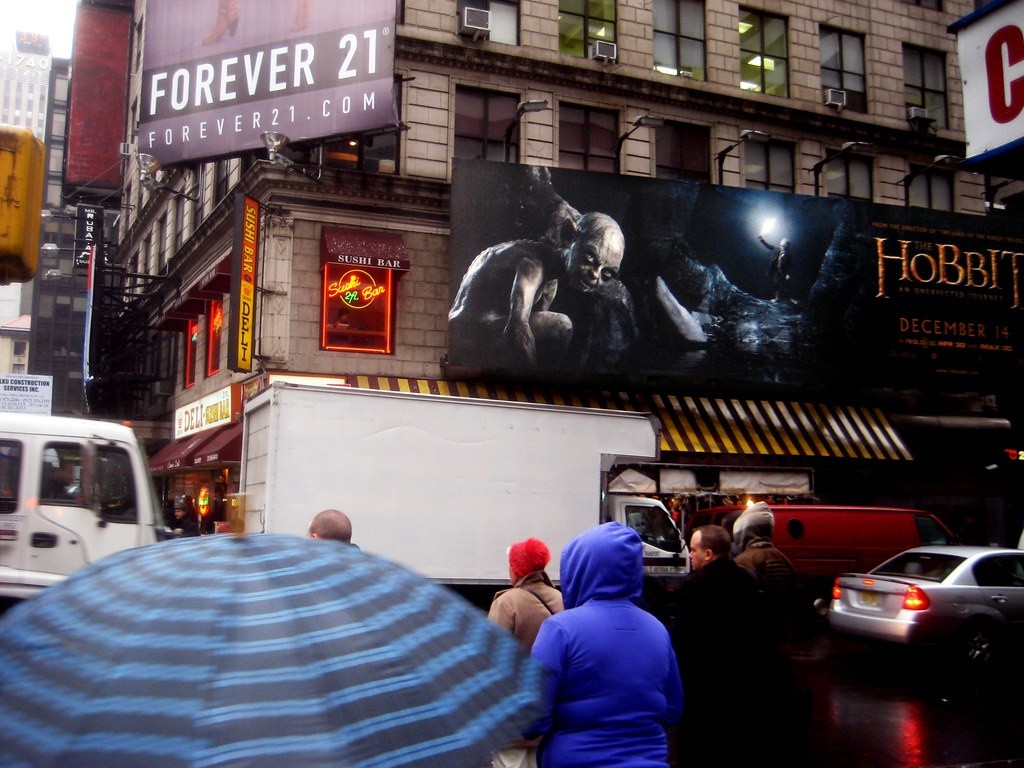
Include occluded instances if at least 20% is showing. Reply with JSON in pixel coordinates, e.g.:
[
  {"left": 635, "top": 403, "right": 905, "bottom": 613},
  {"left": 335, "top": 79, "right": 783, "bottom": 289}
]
[{"left": 508, "top": 539, "right": 550, "bottom": 577}]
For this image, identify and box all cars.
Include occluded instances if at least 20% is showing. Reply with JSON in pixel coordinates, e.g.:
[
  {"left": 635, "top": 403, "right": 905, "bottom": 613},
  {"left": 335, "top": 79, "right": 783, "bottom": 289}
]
[{"left": 826, "top": 544, "right": 1024, "bottom": 670}]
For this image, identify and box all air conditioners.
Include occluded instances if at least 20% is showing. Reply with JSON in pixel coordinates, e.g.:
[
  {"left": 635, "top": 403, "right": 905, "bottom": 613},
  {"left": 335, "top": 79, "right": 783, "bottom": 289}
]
[
  {"left": 132, "top": 120, "right": 138, "bottom": 136},
  {"left": 119, "top": 143, "right": 134, "bottom": 159},
  {"left": 590, "top": 40, "right": 617, "bottom": 63},
  {"left": 908, "top": 107, "right": 928, "bottom": 119},
  {"left": 459, "top": 7, "right": 492, "bottom": 38},
  {"left": 825, "top": 89, "right": 848, "bottom": 109}
]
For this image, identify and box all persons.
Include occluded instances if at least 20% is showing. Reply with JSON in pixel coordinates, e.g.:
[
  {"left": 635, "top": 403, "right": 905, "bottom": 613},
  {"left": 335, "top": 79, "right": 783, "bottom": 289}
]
[
  {"left": 173, "top": 501, "right": 200, "bottom": 538},
  {"left": 661, "top": 501, "right": 797, "bottom": 768},
  {"left": 202, "top": 0, "right": 312, "bottom": 46},
  {"left": 451, "top": 211, "right": 625, "bottom": 369},
  {"left": 516, "top": 522, "right": 684, "bottom": 768},
  {"left": 306, "top": 509, "right": 359, "bottom": 550},
  {"left": 487, "top": 541, "right": 563, "bottom": 658}
]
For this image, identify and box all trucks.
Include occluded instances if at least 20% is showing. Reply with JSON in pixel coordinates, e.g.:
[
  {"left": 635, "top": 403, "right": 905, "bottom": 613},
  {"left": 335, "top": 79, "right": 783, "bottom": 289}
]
[{"left": 236, "top": 380, "right": 692, "bottom": 616}]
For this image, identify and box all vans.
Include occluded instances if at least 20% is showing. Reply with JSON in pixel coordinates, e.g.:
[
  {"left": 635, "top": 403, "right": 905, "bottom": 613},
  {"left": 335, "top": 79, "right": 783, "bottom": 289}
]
[
  {"left": 0, "top": 411, "right": 183, "bottom": 604},
  {"left": 681, "top": 504, "right": 965, "bottom": 629}
]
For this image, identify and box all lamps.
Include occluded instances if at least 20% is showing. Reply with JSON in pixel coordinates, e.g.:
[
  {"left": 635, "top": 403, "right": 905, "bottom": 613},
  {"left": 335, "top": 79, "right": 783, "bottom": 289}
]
[
  {"left": 615, "top": 114, "right": 665, "bottom": 173},
  {"left": 41, "top": 242, "right": 112, "bottom": 256},
  {"left": 41, "top": 209, "right": 107, "bottom": 225},
  {"left": 714, "top": 129, "right": 769, "bottom": 186},
  {"left": 135, "top": 153, "right": 201, "bottom": 204},
  {"left": 895, "top": 154, "right": 958, "bottom": 205},
  {"left": 260, "top": 130, "right": 325, "bottom": 185},
  {"left": 503, "top": 99, "right": 550, "bottom": 163},
  {"left": 44, "top": 268, "right": 88, "bottom": 281},
  {"left": 808, "top": 141, "right": 871, "bottom": 196}
]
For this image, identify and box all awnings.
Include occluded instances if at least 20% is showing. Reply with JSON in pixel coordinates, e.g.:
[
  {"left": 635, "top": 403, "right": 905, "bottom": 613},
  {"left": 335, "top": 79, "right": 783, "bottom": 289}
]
[
  {"left": 147, "top": 423, "right": 243, "bottom": 472},
  {"left": 345, "top": 373, "right": 913, "bottom": 459}
]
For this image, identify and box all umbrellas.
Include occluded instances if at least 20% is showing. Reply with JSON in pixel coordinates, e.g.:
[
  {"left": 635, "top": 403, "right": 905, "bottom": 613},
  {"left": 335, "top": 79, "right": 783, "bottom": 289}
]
[{"left": 0, "top": 531, "right": 551, "bottom": 768}]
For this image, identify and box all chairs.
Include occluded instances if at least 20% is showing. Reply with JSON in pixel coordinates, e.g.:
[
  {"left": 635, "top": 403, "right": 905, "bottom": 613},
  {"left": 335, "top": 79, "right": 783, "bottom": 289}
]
[{"left": 905, "top": 562, "right": 926, "bottom": 576}]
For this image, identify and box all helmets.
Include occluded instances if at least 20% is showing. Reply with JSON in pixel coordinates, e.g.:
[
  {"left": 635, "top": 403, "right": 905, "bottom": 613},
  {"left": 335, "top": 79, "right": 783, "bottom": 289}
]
[{"left": 174, "top": 502, "right": 189, "bottom": 512}]
